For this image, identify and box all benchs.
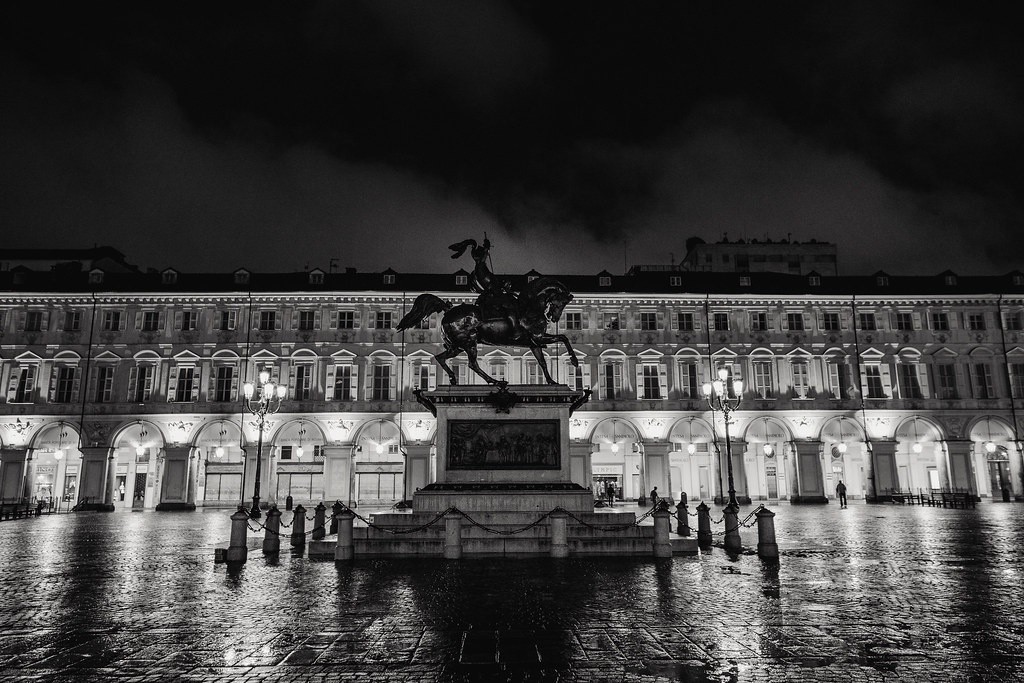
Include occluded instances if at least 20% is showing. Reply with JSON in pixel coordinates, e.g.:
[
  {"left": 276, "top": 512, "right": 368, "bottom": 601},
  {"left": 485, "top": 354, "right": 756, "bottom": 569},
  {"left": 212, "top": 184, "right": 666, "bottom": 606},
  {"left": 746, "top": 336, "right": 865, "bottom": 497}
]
[
  {"left": 892, "top": 491, "right": 976, "bottom": 509},
  {"left": 0, "top": 501, "right": 54, "bottom": 521}
]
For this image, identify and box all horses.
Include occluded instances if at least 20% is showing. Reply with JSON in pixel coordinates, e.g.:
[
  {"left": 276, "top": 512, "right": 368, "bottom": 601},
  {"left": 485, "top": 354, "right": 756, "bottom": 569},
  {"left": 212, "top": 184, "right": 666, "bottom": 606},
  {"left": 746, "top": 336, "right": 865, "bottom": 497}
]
[{"left": 395, "top": 275, "right": 579, "bottom": 386}]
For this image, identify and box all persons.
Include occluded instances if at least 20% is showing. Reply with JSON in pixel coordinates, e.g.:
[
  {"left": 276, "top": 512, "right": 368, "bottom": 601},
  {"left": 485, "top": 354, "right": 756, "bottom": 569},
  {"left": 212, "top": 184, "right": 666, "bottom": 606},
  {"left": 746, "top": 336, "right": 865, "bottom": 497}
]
[
  {"left": 596, "top": 478, "right": 616, "bottom": 499},
  {"left": 651, "top": 486, "right": 659, "bottom": 511},
  {"left": 606, "top": 484, "right": 614, "bottom": 507},
  {"left": 837, "top": 480, "right": 847, "bottom": 509},
  {"left": 469, "top": 243, "right": 529, "bottom": 342}
]
